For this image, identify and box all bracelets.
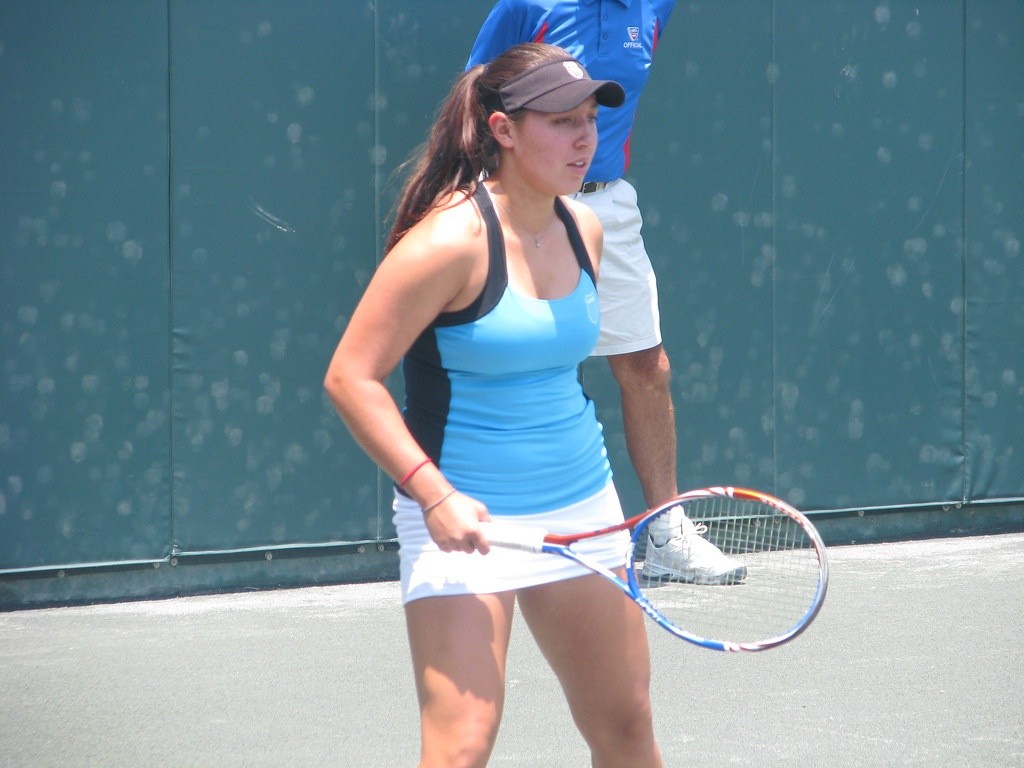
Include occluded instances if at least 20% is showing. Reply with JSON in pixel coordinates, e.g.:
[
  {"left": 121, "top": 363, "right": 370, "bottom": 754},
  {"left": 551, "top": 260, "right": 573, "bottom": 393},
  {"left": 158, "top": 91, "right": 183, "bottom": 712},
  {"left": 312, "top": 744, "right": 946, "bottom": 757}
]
[
  {"left": 401, "top": 458, "right": 432, "bottom": 486},
  {"left": 422, "top": 488, "right": 455, "bottom": 511}
]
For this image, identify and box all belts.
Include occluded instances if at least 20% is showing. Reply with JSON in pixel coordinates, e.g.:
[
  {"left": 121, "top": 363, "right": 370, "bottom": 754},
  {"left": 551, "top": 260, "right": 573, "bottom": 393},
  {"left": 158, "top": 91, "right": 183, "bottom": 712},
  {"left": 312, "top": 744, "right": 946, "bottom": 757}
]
[{"left": 579, "top": 182, "right": 609, "bottom": 194}]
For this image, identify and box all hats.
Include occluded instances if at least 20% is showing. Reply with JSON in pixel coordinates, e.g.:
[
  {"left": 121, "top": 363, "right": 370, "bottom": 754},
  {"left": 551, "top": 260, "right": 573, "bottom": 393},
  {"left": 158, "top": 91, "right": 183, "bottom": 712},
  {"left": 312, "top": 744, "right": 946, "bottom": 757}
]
[{"left": 482, "top": 56, "right": 626, "bottom": 114}]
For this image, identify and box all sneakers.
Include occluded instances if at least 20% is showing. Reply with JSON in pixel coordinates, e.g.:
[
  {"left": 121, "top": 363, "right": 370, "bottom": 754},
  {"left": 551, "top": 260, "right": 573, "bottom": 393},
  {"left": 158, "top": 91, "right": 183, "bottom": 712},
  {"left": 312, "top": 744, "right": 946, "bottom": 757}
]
[{"left": 642, "top": 515, "right": 747, "bottom": 585}]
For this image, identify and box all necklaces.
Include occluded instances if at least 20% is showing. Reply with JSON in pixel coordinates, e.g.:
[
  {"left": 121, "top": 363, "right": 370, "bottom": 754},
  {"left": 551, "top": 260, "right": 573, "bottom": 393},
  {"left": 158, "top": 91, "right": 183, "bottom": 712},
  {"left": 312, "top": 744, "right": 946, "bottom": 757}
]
[{"left": 485, "top": 183, "right": 556, "bottom": 248}]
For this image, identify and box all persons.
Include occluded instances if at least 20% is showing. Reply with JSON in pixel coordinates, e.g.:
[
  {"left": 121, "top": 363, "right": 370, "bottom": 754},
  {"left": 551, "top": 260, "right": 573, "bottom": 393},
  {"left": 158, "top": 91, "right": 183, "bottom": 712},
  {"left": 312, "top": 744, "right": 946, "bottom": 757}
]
[
  {"left": 465, "top": 0, "right": 746, "bottom": 585},
  {"left": 323, "top": 42, "right": 661, "bottom": 768}
]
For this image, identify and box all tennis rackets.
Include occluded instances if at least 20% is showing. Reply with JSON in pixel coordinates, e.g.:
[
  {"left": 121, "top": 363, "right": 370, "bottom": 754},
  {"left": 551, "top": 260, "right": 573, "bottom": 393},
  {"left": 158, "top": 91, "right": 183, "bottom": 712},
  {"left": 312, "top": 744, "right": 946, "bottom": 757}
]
[{"left": 476, "top": 485, "right": 830, "bottom": 653}]
